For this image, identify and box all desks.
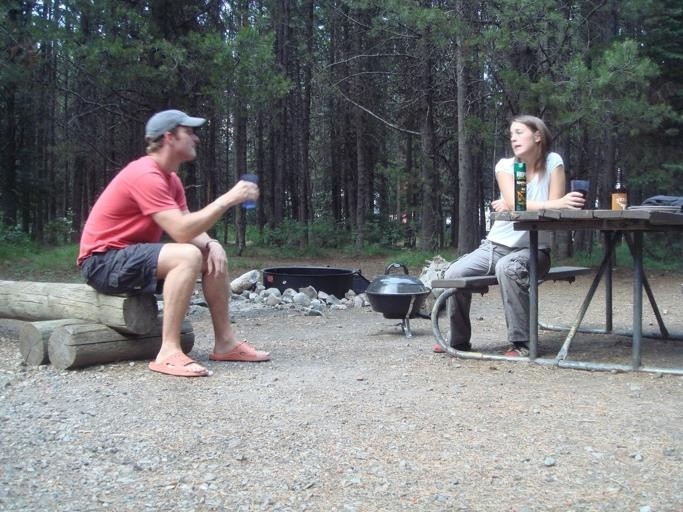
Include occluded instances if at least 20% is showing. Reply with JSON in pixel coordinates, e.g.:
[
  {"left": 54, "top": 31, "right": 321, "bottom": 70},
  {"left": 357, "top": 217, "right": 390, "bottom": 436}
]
[{"left": 491, "top": 211, "right": 683, "bottom": 376}]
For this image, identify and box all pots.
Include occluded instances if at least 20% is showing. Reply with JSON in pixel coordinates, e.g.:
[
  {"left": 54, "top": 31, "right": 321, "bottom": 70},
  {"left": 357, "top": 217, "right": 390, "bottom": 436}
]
[
  {"left": 259, "top": 264, "right": 361, "bottom": 300},
  {"left": 364, "top": 262, "right": 432, "bottom": 319}
]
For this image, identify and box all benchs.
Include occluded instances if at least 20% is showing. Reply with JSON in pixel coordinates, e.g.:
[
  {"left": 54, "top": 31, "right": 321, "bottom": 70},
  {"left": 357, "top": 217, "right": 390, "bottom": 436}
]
[{"left": 431, "top": 266, "right": 592, "bottom": 376}]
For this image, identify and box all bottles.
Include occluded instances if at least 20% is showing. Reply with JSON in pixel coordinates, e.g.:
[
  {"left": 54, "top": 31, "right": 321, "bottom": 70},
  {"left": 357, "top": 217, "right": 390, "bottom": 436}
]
[
  {"left": 513, "top": 161, "right": 527, "bottom": 211},
  {"left": 610, "top": 167, "right": 631, "bottom": 209}
]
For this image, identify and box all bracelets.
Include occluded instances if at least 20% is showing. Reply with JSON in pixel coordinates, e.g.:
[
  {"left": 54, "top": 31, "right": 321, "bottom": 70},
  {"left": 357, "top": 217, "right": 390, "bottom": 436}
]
[{"left": 205, "top": 239, "right": 219, "bottom": 250}]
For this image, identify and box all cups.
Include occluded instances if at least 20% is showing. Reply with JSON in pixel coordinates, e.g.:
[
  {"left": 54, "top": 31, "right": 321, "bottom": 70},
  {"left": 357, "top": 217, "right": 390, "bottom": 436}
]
[
  {"left": 570, "top": 178, "right": 590, "bottom": 210},
  {"left": 239, "top": 173, "right": 259, "bottom": 209}
]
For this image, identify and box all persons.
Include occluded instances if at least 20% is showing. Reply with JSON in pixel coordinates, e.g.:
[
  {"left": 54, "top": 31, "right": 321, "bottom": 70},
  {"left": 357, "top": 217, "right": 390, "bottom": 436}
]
[
  {"left": 76, "top": 108, "right": 272, "bottom": 376},
  {"left": 430, "top": 114, "right": 588, "bottom": 360}
]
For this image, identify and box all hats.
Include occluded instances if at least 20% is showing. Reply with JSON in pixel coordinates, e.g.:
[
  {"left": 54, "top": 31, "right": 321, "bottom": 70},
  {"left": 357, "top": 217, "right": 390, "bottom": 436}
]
[{"left": 145, "top": 109, "right": 206, "bottom": 140}]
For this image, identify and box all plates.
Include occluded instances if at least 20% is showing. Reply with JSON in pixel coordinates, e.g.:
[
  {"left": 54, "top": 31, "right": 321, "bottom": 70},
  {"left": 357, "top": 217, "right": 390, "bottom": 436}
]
[{"left": 626, "top": 204, "right": 682, "bottom": 214}]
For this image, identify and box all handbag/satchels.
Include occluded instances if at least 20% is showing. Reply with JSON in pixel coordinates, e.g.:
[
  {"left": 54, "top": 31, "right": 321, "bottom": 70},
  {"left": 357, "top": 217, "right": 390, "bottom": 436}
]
[{"left": 418, "top": 256, "right": 452, "bottom": 318}]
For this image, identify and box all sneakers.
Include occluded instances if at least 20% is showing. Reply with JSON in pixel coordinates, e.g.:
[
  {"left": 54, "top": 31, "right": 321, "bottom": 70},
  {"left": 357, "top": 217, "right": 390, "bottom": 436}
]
[
  {"left": 505, "top": 343, "right": 528, "bottom": 356},
  {"left": 433, "top": 343, "right": 470, "bottom": 352}
]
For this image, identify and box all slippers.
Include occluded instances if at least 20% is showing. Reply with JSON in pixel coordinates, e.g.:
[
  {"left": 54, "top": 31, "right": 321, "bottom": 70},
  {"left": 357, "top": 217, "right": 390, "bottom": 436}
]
[
  {"left": 149, "top": 352, "right": 208, "bottom": 377},
  {"left": 209, "top": 342, "right": 271, "bottom": 361}
]
